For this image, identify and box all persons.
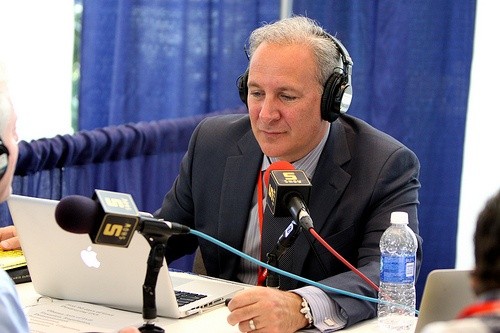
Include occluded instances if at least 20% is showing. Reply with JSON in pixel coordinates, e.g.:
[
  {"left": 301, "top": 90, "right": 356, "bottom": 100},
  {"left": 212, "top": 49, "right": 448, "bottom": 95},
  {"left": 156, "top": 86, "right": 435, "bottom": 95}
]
[
  {"left": 1, "top": 89, "right": 145, "bottom": 333},
  {"left": 1, "top": 15, "right": 424, "bottom": 333},
  {"left": 416, "top": 187, "right": 500, "bottom": 333}
]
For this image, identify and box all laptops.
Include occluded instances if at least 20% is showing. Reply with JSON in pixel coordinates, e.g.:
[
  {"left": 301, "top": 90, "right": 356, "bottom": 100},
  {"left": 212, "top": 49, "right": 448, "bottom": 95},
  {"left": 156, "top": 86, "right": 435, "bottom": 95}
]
[
  {"left": 7, "top": 194, "right": 244, "bottom": 319},
  {"left": 414, "top": 269, "right": 474, "bottom": 333}
]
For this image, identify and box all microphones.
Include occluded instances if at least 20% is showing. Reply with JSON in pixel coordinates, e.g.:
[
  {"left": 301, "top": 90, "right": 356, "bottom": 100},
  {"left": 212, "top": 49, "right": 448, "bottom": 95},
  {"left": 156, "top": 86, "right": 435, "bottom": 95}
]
[
  {"left": 264, "top": 161, "right": 315, "bottom": 232},
  {"left": 54, "top": 189, "right": 190, "bottom": 248}
]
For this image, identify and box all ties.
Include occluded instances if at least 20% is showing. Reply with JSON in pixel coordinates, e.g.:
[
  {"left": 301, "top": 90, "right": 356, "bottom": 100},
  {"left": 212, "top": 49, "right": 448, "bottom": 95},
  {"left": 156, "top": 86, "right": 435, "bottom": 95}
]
[{"left": 258, "top": 170, "right": 293, "bottom": 291}]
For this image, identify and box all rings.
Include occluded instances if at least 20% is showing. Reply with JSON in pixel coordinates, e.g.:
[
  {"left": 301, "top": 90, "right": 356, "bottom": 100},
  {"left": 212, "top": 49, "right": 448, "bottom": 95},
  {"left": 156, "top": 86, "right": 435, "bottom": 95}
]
[{"left": 249, "top": 320, "right": 256, "bottom": 331}]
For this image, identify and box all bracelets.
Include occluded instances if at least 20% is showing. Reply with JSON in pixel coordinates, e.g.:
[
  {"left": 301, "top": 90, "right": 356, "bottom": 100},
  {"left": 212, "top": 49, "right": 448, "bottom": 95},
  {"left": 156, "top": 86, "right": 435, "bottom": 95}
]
[{"left": 299, "top": 296, "right": 317, "bottom": 331}]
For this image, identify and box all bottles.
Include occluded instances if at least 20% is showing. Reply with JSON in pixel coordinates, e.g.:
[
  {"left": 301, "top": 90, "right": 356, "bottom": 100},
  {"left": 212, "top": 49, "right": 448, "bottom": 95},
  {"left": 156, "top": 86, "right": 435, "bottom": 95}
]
[{"left": 378, "top": 211, "right": 419, "bottom": 333}]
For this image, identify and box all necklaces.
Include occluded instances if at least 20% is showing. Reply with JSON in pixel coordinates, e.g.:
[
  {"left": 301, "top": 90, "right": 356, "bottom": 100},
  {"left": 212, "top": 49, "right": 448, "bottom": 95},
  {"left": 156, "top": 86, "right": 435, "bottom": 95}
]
[
  {"left": 456, "top": 299, "right": 500, "bottom": 319},
  {"left": 255, "top": 168, "right": 268, "bottom": 287}
]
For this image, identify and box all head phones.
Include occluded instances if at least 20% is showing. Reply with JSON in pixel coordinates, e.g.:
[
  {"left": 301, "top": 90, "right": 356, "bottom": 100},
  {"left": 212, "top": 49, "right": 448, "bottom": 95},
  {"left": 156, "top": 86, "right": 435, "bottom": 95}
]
[{"left": 237, "top": 31, "right": 353, "bottom": 123}]
